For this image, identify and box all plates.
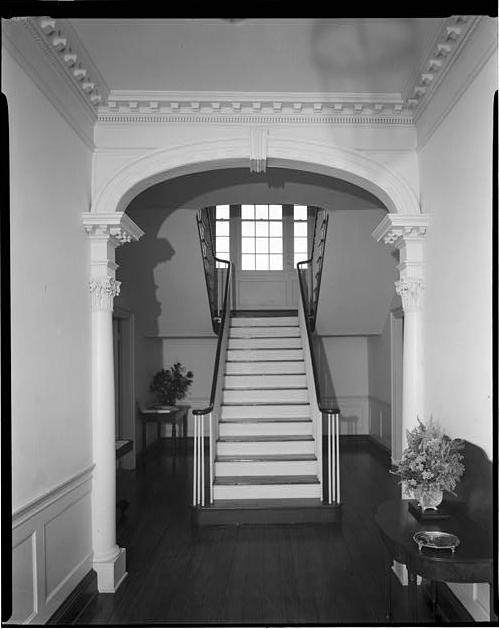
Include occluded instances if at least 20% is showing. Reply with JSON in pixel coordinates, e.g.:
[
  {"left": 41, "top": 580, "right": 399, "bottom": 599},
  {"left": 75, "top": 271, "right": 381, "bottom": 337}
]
[{"left": 413, "top": 531, "right": 461, "bottom": 549}]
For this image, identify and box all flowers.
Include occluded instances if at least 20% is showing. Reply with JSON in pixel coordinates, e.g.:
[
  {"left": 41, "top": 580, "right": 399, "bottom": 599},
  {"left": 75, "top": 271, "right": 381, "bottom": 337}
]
[{"left": 388, "top": 414, "right": 466, "bottom": 498}]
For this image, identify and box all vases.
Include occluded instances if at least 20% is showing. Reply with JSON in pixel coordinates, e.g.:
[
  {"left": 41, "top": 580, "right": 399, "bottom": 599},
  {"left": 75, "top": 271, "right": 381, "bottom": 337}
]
[{"left": 411, "top": 487, "right": 442, "bottom": 513}]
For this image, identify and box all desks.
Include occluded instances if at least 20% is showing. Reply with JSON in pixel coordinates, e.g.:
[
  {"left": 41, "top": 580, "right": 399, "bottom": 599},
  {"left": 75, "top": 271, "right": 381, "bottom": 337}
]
[
  {"left": 373, "top": 439, "right": 492, "bottom": 621},
  {"left": 138, "top": 406, "right": 190, "bottom": 470}
]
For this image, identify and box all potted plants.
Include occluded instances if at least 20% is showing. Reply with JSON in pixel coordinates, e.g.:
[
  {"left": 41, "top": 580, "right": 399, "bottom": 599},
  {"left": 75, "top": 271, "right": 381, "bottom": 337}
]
[{"left": 149, "top": 360, "right": 197, "bottom": 403}]
